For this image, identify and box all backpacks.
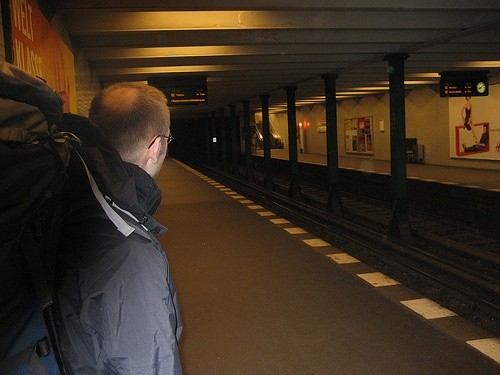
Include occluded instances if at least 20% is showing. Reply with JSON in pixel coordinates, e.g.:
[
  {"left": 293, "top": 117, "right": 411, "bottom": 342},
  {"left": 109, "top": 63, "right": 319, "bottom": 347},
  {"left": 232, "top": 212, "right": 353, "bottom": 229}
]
[{"left": 1, "top": 62, "right": 151, "bottom": 372}]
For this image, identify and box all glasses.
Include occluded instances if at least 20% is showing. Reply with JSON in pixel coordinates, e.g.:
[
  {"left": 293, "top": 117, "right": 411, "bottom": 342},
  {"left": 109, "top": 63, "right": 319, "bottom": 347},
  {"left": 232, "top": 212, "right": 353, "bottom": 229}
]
[{"left": 148, "top": 134, "right": 172, "bottom": 148}]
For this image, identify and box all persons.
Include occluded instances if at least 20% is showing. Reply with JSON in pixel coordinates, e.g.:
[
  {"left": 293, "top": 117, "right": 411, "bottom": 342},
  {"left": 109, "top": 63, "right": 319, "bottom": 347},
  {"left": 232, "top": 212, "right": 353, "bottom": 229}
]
[{"left": 0, "top": 85, "right": 184, "bottom": 375}]
